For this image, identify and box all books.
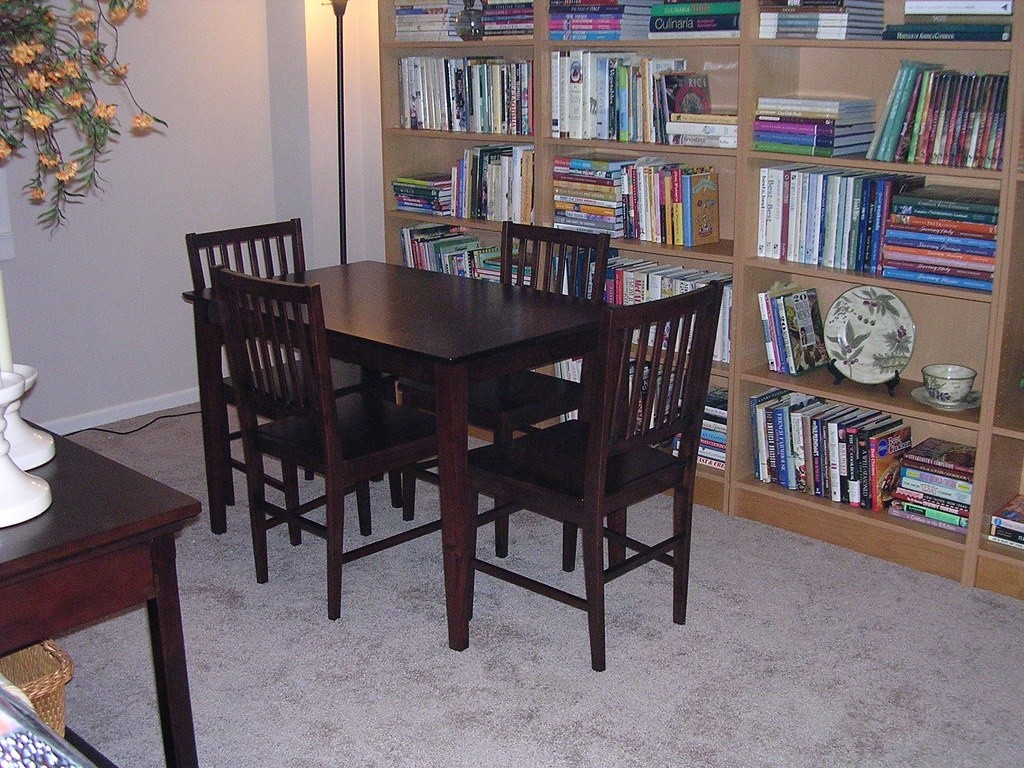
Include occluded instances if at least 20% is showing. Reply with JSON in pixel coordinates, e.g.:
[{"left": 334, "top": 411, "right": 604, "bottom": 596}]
[
  {"left": 756, "top": 280, "right": 830, "bottom": 376},
  {"left": 555, "top": 352, "right": 728, "bottom": 470},
  {"left": 551, "top": 49, "right": 737, "bottom": 149},
  {"left": 751, "top": 59, "right": 1009, "bottom": 171},
  {"left": 756, "top": 0, "right": 1014, "bottom": 41},
  {"left": 548, "top": 0, "right": 740, "bottom": 42},
  {"left": 552, "top": 150, "right": 722, "bottom": 248},
  {"left": 397, "top": 55, "right": 534, "bottom": 138},
  {"left": 399, "top": 221, "right": 532, "bottom": 287},
  {"left": 749, "top": 387, "right": 977, "bottom": 537},
  {"left": 393, "top": 0, "right": 533, "bottom": 42},
  {"left": 755, "top": 161, "right": 999, "bottom": 293},
  {"left": 552, "top": 248, "right": 732, "bottom": 364},
  {"left": 391, "top": 143, "right": 535, "bottom": 225},
  {"left": 987, "top": 493, "right": 1024, "bottom": 550}
]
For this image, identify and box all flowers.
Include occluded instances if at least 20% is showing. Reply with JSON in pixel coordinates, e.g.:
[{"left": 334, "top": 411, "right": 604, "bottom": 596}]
[{"left": 1, "top": 0, "right": 170, "bottom": 238}]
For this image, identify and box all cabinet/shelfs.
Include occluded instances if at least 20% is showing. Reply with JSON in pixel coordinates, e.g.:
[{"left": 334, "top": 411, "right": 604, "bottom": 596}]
[{"left": 378, "top": 0, "right": 1024, "bottom": 602}]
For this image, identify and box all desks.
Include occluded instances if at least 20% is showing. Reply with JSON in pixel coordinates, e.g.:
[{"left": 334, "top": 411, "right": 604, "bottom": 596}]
[{"left": 0, "top": 418, "right": 205, "bottom": 768}]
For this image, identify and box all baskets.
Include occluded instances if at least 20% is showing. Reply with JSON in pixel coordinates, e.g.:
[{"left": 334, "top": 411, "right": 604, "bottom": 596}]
[{"left": 0, "top": 640, "right": 73, "bottom": 738}]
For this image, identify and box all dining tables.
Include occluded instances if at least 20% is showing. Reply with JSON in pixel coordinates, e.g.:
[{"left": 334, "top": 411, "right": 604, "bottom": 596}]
[{"left": 182, "top": 261, "right": 636, "bottom": 653}]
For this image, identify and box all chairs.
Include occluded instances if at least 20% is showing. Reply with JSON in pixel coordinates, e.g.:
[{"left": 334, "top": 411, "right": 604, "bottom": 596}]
[
  {"left": 458, "top": 277, "right": 726, "bottom": 672},
  {"left": 211, "top": 263, "right": 435, "bottom": 621},
  {"left": 403, "top": 219, "right": 611, "bottom": 559},
  {"left": 185, "top": 216, "right": 403, "bottom": 509}
]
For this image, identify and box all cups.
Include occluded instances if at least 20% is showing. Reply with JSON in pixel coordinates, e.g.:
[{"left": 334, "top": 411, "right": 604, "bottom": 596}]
[{"left": 456, "top": 11, "right": 484, "bottom": 40}]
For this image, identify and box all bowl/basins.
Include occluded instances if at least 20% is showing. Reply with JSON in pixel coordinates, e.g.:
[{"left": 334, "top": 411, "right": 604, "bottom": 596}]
[{"left": 921, "top": 364, "right": 977, "bottom": 405}]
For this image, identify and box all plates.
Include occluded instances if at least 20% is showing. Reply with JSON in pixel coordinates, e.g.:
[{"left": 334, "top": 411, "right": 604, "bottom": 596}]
[{"left": 911, "top": 387, "right": 982, "bottom": 412}]
